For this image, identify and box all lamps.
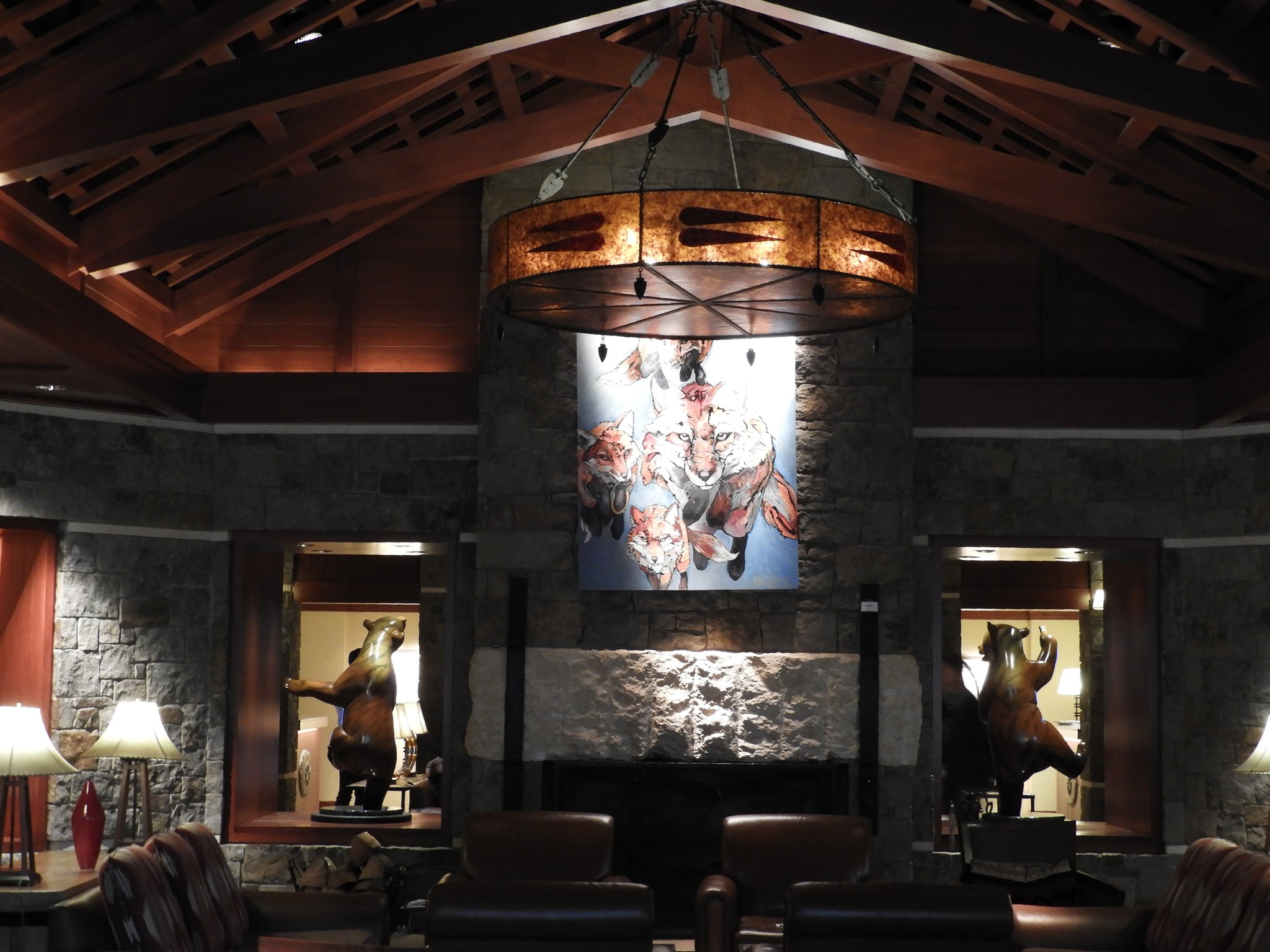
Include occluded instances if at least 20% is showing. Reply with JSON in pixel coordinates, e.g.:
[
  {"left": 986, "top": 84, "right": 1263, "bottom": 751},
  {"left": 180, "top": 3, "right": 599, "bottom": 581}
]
[
  {"left": 79, "top": 701, "right": 184, "bottom": 847},
  {"left": 0, "top": 702, "right": 81, "bottom": 886},
  {"left": 393, "top": 701, "right": 429, "bottom": 779}
]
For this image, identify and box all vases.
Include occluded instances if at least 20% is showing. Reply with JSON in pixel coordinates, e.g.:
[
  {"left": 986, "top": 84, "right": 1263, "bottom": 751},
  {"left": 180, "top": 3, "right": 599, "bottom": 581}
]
[{"left": 71, "top": 780, "right": 105, "bottom": 870}]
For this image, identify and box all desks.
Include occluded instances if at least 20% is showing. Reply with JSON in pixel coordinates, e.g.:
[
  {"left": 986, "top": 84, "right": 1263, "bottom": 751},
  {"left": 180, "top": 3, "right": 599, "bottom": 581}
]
[
  {"left": 0, "top": 848, "right": 112, "bottom": 952},
  {"left": 345, "top": 774, "right": 427, "bottom": 812}
]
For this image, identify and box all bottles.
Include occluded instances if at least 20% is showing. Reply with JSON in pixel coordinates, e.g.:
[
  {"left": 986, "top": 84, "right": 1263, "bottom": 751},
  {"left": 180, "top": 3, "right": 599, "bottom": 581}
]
[{"left": 71, "top": 781, "right": 106, "bottom": 870}]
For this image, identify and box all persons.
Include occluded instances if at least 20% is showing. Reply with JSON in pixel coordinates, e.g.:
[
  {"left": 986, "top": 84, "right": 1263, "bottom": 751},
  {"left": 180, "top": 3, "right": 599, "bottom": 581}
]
[
  {"left": 334, "top": 648, "right": 366, "bottom": 805},
  {"left": 940, "top": 654, "right": 993, "bottom": 885}
]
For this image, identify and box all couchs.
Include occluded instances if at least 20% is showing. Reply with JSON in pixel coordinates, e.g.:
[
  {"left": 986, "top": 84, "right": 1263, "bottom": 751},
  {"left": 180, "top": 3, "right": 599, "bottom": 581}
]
[{"left": 101, "top": 810, "right": 1270, "bottom": 952}]
